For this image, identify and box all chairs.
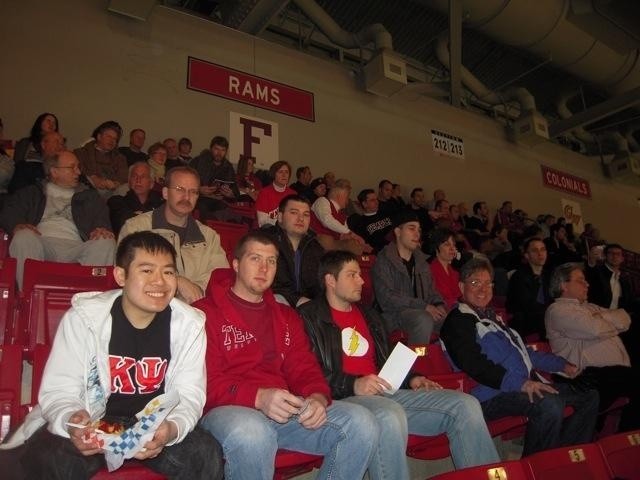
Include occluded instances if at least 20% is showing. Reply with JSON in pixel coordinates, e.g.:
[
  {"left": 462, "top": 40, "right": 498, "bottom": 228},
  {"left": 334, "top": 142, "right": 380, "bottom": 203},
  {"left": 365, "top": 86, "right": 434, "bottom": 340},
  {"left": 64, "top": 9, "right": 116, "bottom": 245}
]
[{"left": 1, "top": 139, "right": 640, "bottom": 479}]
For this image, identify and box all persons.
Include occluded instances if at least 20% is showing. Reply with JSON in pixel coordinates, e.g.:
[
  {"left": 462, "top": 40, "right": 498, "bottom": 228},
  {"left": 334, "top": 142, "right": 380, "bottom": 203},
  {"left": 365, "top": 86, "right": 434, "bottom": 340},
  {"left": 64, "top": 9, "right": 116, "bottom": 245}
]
[{"left": 1, "top": 113, "right": 640, "bottom": 479}]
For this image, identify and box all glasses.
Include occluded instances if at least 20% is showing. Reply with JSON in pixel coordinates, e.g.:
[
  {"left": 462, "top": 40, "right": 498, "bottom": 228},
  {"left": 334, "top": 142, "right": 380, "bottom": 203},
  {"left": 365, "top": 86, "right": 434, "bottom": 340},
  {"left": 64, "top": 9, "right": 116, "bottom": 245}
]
[
  {"left": 52, "top": 165, "right": 80, "bottom": 169},
  {"left": 168, "top": 186, "right": 199, "bottom": 196},
  {"left": 471, "top": 279, "right": 495, "bottom": 289}
]
